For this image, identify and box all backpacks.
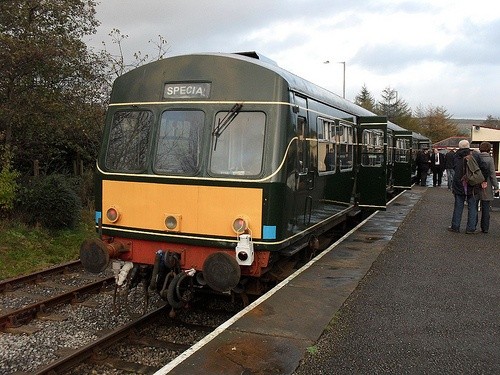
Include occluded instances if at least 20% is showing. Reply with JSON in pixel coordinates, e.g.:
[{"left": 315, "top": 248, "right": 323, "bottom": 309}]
[{"left": 464, "top": 155, "right": 485, "bottom": 185}]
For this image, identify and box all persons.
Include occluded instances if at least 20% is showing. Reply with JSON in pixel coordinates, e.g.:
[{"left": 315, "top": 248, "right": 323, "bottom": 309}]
[{"left": 418, "top": 140, "right": 500, "bottom": 233}]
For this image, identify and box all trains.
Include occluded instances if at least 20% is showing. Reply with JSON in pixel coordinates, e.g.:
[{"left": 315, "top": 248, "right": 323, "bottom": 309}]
[{"left": 94, "top": 51, "right": 432, "bottom": 320}]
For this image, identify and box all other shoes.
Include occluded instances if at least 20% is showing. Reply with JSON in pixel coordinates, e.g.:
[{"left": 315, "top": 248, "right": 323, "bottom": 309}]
[
  {"left": 482, "top": 230, "right": 488, "bottom": 233},
  {"left": 448, "top": 228, "right": 459, "bottom": 232},
  {"left": 466, "top": 230, "right": 478, "bottom": 233}
]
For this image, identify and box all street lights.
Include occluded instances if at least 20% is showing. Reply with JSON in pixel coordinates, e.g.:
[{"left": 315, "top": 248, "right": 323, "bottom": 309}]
[{"left": 323, "top": 60, "right": 345, "bottom": 99}]
